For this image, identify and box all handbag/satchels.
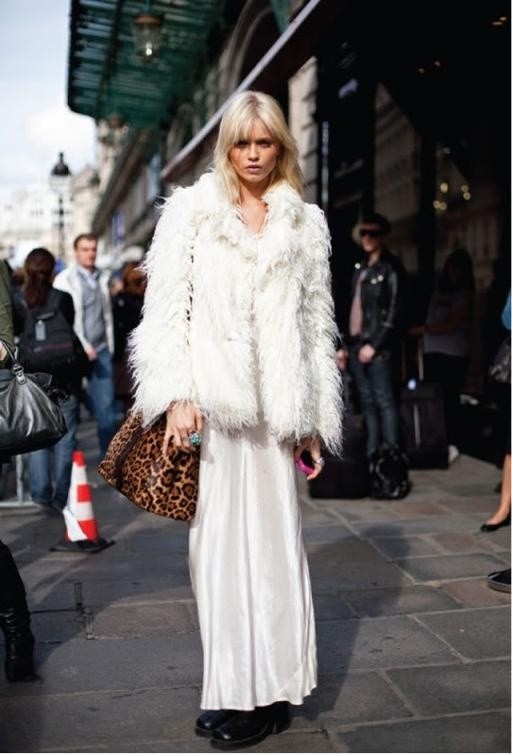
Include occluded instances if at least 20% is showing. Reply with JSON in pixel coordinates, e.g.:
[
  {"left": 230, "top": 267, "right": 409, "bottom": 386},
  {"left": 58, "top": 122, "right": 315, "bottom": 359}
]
[
  {"left": 1, "top": 340, "right": 68, "bottom": 454},
  {"left": 367, "top": 442, "right": 410, "bottom": 499},
  {"left": 486, "top": 335, "right": 511, "bottom": 384},
  {"left": 97, "top": 404, "right": 198, "bottom": 521}
]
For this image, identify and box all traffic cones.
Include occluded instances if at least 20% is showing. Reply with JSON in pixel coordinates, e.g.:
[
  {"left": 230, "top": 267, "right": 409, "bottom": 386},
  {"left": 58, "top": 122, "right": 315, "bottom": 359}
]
[{"left": 51, "top": 450, "right": 116, "bottom": 554}]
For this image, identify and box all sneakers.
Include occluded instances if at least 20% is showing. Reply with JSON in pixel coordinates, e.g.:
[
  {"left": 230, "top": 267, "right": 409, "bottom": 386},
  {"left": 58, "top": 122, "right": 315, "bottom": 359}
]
[{"left": 487, "top": 568, "right": 511, "bottom": 592}]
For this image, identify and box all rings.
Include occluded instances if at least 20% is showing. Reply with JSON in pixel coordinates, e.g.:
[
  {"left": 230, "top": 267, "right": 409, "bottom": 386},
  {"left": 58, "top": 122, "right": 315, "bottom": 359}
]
[
  {"left": 313, "top": 457, "right": 325, "bottom": 468},
  {"left": 188, "top": 428, "right": 203, "bottom": 446}
]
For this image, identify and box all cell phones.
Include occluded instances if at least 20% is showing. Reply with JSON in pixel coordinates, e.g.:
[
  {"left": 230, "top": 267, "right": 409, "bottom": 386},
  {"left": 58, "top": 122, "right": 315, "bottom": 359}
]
[{"left": 294, "top": 446, "right": 315, "bottom": 475}]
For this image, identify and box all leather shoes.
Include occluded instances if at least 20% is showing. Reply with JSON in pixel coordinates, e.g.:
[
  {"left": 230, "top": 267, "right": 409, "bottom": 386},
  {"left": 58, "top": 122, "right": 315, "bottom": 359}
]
[
  {"left": 194, "top": 699, "right": 288, "bottom": 750},
  {"left": 480, "top": 512, "right": 510, "bottom": 531}
]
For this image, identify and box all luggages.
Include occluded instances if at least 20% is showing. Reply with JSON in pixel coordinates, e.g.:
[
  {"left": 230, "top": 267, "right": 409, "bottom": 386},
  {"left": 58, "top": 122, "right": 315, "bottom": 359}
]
[
  {"left": 306, "top": 381, "right": 369, "bottom": 498},
  {"left": 399, "top": 333, "right": 449, "bottom": 469}
]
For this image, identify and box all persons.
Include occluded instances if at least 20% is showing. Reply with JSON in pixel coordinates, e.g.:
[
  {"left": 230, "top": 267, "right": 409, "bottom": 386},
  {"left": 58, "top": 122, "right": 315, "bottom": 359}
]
[
  {"left": 2, "top": 260, "right": 34, "bottom": 682},
  {"left": 480, "top": 295, "right": 510, "bottom": 589},
  {"left": 119, "top": 90, "right": 346, "bottom": 752},
  {"left": 15, "top": 206, "right": 475, "bottom": 512}
]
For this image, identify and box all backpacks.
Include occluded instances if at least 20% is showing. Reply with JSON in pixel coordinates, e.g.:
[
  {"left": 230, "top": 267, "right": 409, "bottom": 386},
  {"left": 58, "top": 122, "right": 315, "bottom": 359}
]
[{"left": 18, "top": 290, "right": 77, "bottom": 370}]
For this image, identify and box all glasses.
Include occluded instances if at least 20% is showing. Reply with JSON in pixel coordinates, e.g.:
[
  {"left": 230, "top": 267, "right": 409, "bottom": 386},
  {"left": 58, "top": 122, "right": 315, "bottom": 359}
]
[{"left": 359, "top": 229, "right": 382, "bottom": 238}]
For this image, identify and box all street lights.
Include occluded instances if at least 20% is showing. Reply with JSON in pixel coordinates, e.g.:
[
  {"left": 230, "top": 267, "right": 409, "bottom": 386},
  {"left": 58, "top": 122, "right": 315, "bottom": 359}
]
[{"left": 48, "top": 150, "right": 73, "bottom": 260}]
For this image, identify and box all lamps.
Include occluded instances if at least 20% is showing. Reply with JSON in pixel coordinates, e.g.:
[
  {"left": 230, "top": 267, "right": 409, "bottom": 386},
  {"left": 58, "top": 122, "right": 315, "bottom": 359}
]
[{"left": 127, "top": 1, "right": 169, "bottom": 65}]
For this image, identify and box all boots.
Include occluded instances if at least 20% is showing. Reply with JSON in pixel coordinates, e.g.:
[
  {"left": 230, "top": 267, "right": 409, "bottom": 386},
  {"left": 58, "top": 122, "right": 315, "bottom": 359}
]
[{"left": 1, "top": 546, "right": 34, "bottom": 683}]
